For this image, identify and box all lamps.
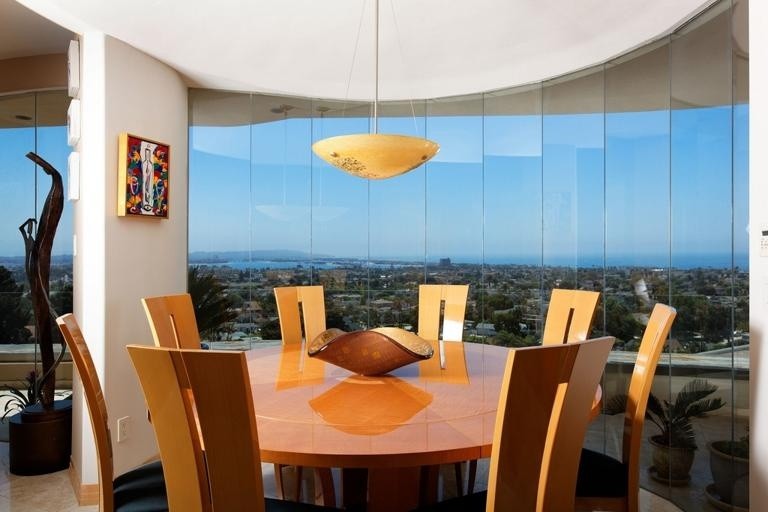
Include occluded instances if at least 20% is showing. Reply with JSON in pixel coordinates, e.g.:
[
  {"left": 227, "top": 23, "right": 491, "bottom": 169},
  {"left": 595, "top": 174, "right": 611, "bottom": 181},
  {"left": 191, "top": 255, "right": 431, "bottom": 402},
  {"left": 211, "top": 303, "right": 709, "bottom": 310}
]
[{"left": 311, "top": 0, "right": 441, "bottom": 181}]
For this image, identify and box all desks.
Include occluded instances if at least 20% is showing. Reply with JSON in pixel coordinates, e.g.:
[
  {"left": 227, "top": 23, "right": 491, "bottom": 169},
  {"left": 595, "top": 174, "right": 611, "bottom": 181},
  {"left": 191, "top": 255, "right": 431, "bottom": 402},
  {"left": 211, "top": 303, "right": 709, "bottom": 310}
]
[{"left": 191, "top": 339, "right": 603, "bottom": 512}]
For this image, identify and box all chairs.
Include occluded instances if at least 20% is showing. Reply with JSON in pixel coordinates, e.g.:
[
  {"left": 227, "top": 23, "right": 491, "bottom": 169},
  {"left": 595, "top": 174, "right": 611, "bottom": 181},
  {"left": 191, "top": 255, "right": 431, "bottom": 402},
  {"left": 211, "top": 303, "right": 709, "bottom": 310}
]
[
  {"left": 55, "top": 311, "right": 170, "bottom": 512},
  {"left": 275, "top": 342, "right": 324, "bottom": 391},
  {"left": 405, "top": 334, "right": 618, "bottom": 512},
  {"left": 576, "top": 301, "right": 678, "bottom": 512},
  {"left": 541, "top": 288, "right": 601, "bottom": 346},
  {"left": 419, "top": 341, "right": 469, "bottom": 384},
  {"left": 418, "top": 284, "right": 469, "bottom": 341},
  {"left": 141, "top": 293, "right": 202, "bottom": 349},
  {"left": 126, "top": 345, "right": 344, "bottom": 512},
  {"left": 273, "top": 285, "right": 327, "bottom": 342}
]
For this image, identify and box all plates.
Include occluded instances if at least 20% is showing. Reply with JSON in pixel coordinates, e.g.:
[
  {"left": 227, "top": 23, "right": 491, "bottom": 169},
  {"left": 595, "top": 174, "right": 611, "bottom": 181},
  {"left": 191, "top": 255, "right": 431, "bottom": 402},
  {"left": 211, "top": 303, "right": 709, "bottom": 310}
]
[{"left": 307, "top": 327, "right": 434, "bottom": 376}]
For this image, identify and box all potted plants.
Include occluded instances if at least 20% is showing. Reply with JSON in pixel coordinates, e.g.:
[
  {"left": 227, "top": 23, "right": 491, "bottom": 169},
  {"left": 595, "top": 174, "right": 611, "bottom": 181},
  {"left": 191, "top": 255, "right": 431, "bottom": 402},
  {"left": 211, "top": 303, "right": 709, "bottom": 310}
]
[
  {"left": 600, "top": 378, "right": 728, "bottom": 486},
  {"left": 703, "top": 418, "right": 749, "bottom": 512}
]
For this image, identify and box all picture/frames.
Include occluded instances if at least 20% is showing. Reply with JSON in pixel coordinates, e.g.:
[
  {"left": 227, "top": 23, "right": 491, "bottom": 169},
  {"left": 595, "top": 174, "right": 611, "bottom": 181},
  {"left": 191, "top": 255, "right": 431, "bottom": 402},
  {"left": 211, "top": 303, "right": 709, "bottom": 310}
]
[
  {"left": 68, "top": 151, "right": 80, "bottom": 201},
  {"left": 67, "top": 39, "right": 80, "bottom": 98},
  {"left": 66, "top": 99, "right": 81, "bottom": 146}
]
